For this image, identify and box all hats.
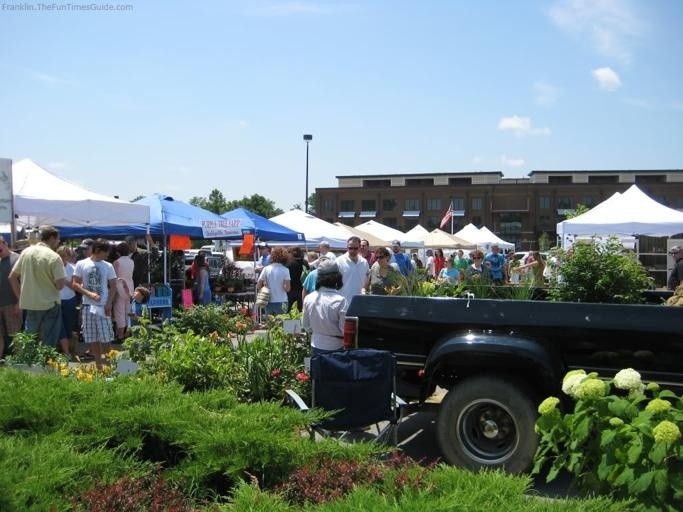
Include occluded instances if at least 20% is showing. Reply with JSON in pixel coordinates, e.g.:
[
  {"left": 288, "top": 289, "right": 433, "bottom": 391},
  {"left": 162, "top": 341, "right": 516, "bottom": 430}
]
[
  {"left": 75, "top": 238, "right": 95, "bottom": 250},
  {"left": 391, "top": 240, "right": 400, "bottom": 247},
  {"left": 316, "top": 259, "right": 340, "bottom": 275}
]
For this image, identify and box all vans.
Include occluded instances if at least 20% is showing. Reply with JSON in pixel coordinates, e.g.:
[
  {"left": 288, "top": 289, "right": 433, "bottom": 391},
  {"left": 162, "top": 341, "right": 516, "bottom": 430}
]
[{"left": 510, "top": 250, "right": 549, "bottom": 268}]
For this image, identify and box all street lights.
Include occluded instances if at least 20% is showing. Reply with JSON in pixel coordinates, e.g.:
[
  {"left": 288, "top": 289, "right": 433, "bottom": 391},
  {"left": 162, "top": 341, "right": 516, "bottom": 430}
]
[{"left": 301, "top": 133, "right": 313, "bottom": 215}]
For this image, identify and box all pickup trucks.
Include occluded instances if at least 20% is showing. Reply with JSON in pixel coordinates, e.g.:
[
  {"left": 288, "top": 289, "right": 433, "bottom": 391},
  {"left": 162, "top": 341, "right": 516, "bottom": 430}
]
[{"left": 340, "top": 289, "right": 682, "bottom": 482}]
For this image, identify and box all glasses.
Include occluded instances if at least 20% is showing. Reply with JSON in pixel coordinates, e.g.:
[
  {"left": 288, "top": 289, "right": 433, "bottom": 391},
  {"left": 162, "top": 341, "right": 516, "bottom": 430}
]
[
  {"left": 347, "top": 245, "right": 359, "bottom": 250},
  {"left": 473, "top": 256, "right": 481, "bottom": 260}
]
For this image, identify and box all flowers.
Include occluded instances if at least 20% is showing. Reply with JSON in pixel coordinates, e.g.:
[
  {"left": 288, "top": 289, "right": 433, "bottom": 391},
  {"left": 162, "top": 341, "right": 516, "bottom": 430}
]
[{"left": 379, "top": 271, "right": 469, "bottom": 301}]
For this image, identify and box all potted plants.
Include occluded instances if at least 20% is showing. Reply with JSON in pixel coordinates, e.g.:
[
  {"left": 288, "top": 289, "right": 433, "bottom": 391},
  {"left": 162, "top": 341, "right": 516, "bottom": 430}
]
[
  {"left": 212, "top": 274, "right": 223, "bottom": 293},
  {"left": 223, "top": 278, "right": 237, "bottom": 293}
]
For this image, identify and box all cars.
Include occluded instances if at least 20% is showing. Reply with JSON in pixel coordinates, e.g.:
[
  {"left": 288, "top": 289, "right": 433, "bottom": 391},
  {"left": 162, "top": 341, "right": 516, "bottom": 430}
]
[{"left": 159, "top": 243, "right": 225, "bottom": 284}]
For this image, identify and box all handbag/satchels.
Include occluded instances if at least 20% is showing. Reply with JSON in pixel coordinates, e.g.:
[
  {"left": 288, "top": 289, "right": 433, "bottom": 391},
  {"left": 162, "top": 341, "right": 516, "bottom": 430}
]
[{"left": 255, "top": 281, "right": 270, "bottom": 308}]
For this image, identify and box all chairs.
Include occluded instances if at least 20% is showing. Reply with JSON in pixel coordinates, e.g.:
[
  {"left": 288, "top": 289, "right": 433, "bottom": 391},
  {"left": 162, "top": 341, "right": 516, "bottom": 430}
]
[{"left": 280, "top": 347, "right": 409, "bottom": 460}]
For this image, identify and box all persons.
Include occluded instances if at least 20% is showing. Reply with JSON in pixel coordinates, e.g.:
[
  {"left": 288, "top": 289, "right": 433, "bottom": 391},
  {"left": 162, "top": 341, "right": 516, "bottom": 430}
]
[{"left": 666, "top": 245, "right": 683, "bottom": 290}]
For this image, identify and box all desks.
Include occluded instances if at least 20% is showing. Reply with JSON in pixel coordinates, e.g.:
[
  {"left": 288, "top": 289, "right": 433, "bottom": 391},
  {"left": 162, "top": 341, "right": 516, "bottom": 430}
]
[
  {"left": 128, "top": 293, "right": 173, "bottom": 340},
  {"left": 223, "top": 290, "right": 255, "bottom": 316}
]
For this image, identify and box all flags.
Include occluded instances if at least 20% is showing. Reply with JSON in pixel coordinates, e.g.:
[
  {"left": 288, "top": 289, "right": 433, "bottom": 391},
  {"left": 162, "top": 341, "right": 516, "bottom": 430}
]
[{"left": 440, "top": 201, "right": 454, "bottom": 229}]
